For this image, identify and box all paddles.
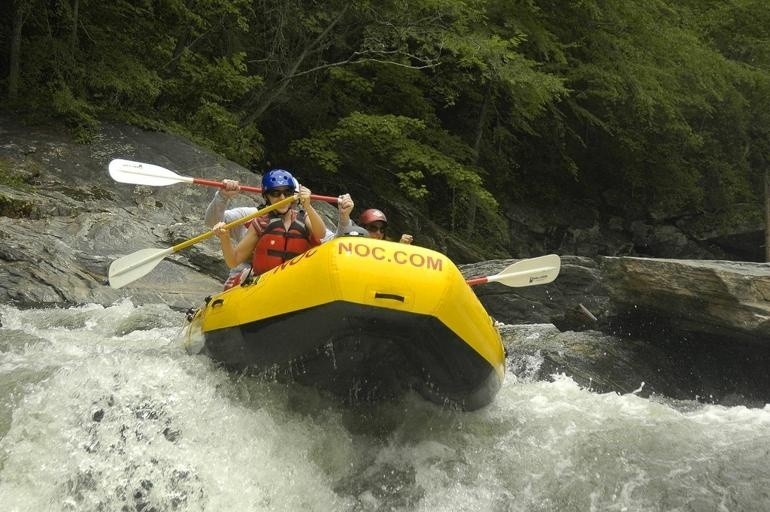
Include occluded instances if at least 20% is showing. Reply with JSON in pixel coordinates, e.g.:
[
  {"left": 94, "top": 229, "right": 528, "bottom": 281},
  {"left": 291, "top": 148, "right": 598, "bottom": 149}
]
[
  {"left": 108, "top": 158, "right": 342, "bottom": 204},
  {"left": 465, "top": 254, "right": 561, "bottom": 287},
  {"left": 108, "top": 194, "right": 300, "bottom": 289}
]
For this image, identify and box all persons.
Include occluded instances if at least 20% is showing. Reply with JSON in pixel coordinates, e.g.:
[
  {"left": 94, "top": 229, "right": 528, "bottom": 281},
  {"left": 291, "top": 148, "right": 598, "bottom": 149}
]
[
  {"left": 212, "top": 168, "right": 326, "bottom": 276},
  {"left": 358, "top": 208, "right": 414, "bottom": 245},
  {"left": 204, "top": 176, "right": 355, "bottom": 293}
]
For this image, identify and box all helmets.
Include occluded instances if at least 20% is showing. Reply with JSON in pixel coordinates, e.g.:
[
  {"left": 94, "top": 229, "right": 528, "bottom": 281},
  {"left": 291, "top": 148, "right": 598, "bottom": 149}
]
[
  {"left": 261, "top": 168, "right": 296, "bottom": 195},
  {"left": 359, "top": 207, "right": 389, "bottom": 227}
]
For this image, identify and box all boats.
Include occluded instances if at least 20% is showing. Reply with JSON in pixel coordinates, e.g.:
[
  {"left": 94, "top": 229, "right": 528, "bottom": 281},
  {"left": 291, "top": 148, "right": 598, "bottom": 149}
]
[{"left": 177, "top": 231, "right": 506, "bottom": 411}]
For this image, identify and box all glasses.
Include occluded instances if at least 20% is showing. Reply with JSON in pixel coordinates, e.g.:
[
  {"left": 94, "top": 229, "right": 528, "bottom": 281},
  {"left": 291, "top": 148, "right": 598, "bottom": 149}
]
[
  {"left": 267, "top": 190, "right": 292, "bottom": 197},
  {"left": 366, "top": 225, "right": 386, "bottom": 233}
]
